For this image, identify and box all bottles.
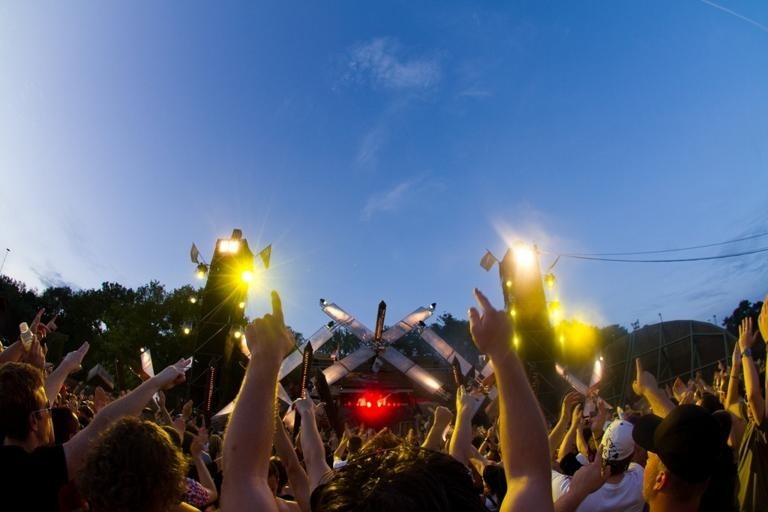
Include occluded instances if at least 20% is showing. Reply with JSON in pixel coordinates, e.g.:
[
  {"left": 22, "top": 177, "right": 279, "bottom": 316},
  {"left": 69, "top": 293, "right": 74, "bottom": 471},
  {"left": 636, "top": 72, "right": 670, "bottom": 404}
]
[{"left": 18, "top": 323, "right": 35, "bottom": 353}]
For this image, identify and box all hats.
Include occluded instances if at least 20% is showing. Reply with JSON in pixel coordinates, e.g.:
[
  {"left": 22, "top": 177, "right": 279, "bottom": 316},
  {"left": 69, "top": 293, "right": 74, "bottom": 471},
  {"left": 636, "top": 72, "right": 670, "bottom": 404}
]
[
  {"left": 632, "top": 404, "right": 713, "bottom": 483},
  {"left": 598, "top": 419, "right": 635, "bottom": 462}
]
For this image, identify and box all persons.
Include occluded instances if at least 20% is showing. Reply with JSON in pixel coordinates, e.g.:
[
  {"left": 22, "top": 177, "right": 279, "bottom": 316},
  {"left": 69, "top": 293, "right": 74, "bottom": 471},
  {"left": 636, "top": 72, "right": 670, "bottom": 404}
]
[{"left": 0, "top": 285, "right": 767, "bottom": 512}]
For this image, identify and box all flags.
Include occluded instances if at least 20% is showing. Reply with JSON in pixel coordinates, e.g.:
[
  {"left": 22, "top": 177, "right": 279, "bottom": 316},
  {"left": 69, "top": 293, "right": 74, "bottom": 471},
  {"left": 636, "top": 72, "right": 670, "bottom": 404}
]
[
  {"left": 260, "top": 245, "right": 272, "bottom": 269},
  {"left": 190, "top": 244, "right": 200, "bottom": 264},
  {"left": 480, "top": 253, "right": 496, "bottom": 272}
]
